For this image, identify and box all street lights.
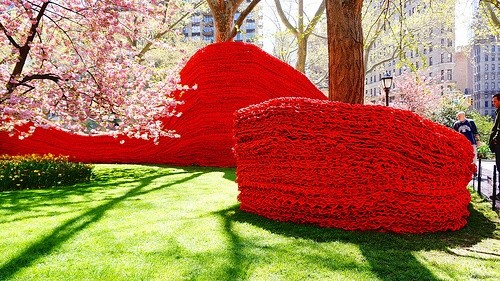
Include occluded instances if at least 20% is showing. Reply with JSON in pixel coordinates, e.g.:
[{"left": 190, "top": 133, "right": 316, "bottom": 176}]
[{"left": 381, "top": 70, "right": 393, "bottom": 108}]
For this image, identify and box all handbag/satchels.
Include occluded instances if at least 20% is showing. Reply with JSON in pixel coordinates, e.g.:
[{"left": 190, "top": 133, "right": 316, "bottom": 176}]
[{"left": 489, "top": 131, "right": 496, "bottom": 153}]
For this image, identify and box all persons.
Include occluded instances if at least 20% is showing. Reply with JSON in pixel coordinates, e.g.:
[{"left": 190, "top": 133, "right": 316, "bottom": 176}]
[
  {"left": 451, "top": 110, "right": 481, "bottom": 178},
  {"left": 488, "top": 93, "right": 499, "bottom": 201}
]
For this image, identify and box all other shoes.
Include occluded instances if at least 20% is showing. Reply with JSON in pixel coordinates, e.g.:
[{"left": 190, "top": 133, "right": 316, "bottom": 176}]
[
  {"left": 472, "top": 173, "right": 478, "bottom": 180},
  {"left": 490, "top": 192, "right": 500, "bottom": 201}
]
